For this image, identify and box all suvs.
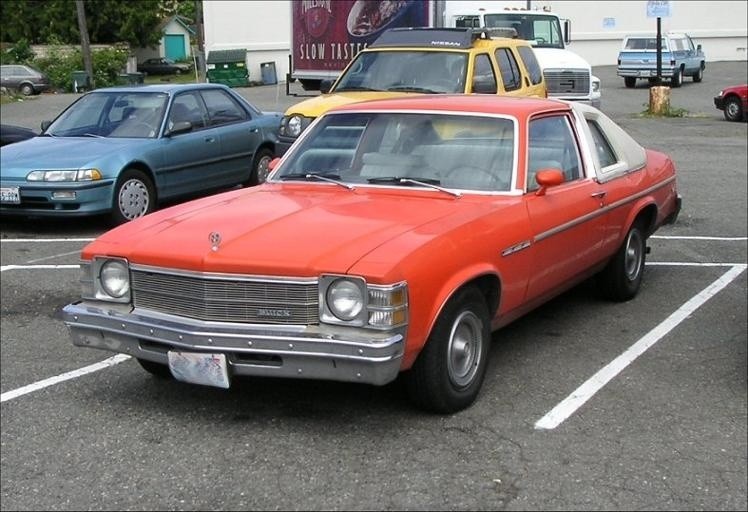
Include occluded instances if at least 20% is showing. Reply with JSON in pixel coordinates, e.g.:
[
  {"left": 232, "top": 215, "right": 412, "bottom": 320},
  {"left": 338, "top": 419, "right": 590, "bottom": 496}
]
[
  {"left": 271, "top": 28, "right": 548, "bottom": 162},
  {"left": 617, "top": 30, "right": 706, "bottom": 90}
]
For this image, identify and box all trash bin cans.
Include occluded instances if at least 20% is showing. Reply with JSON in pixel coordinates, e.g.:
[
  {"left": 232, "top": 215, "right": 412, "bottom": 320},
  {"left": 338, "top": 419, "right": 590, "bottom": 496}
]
[
  {"left": 71, "top": 71, "right": 88, "bottom": 93},
  {"left": 261, "top": 62, "right": 277, "bottom": 85},
  {"left": 119, "top": 72, "right": 144, "bottom": 86}
]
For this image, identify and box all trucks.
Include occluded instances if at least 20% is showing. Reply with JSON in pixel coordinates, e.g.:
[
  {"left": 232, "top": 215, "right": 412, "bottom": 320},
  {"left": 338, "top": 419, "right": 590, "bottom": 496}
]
[{"left": 446, "top": 6, "right": 601, "bottom": 111}]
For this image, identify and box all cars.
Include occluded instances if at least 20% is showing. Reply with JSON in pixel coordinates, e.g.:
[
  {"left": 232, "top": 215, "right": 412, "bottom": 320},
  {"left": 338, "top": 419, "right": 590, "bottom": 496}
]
[
  {"left": 62, "top": 93, "right": 681, "bottom": 415},
  {"left": 713, "top": 84, "right": 748, "bottom": 122},
  {"left": 0, "top": 123, "right": 38, "bottom": 149},
  {"left": 137, "top": 57, "right": 189, "bottom": 77},
  {"left": 1, "top": 65, "right": 50, "bottom": 96},
  {"left": 0, "top": 83, "right": 285, "bottom": 230}
]
